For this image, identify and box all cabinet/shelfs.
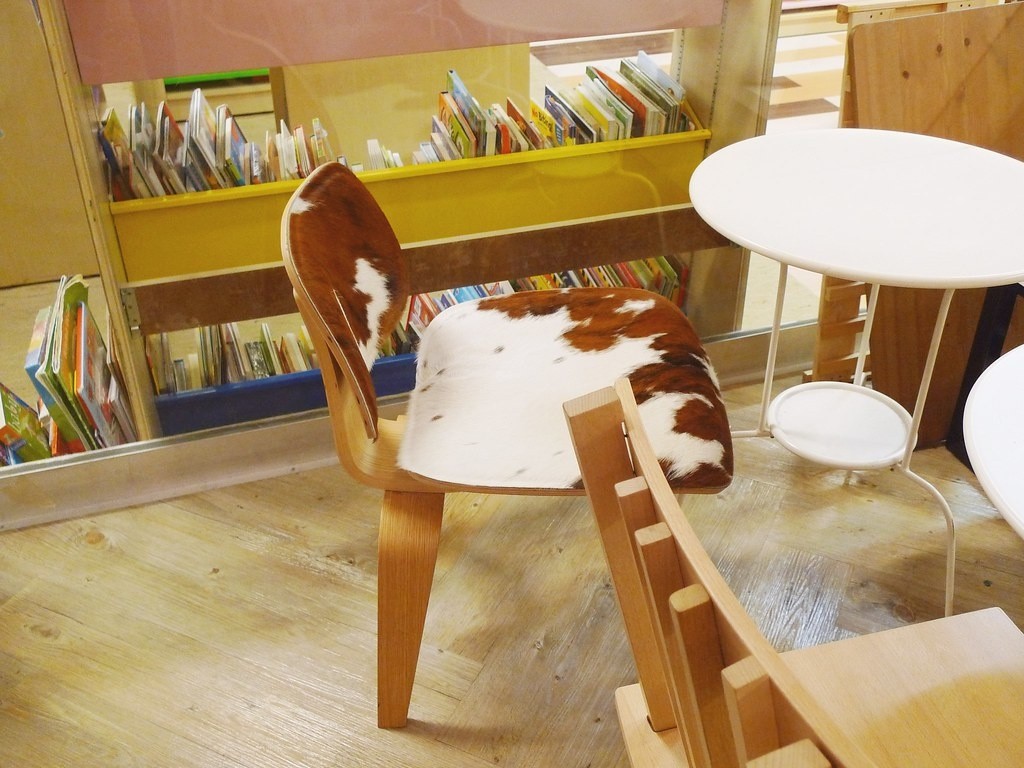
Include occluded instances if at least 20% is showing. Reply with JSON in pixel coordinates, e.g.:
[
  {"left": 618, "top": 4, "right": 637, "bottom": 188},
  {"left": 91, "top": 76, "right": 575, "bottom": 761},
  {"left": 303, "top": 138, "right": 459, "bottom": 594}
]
[{"left": 33, "top": 0, "right": 781, "bottom": 440}]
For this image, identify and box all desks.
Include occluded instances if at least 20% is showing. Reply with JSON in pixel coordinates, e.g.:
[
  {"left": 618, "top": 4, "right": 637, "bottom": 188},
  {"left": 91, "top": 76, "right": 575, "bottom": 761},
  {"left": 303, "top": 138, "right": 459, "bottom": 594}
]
[
  {"left": 963, "top": 343, "right": 1024, "bottom": 540},
  {"left": 672, "top": 128, "right": 1023, "bottom": 619}
]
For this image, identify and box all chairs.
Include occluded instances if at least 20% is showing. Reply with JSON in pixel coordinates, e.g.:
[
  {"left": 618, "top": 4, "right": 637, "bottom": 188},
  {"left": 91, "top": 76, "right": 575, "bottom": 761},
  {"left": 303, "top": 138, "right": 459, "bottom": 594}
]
[
  {"left": 279, "top": 161, "right": 734, "bottom": 729},
  {"left": 562, "top": 376, "right": 1024, "bottom": 768}
]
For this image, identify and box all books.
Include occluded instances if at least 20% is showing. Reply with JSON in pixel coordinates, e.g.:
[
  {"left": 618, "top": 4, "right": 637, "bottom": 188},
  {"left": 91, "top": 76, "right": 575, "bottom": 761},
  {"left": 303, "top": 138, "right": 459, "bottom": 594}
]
[
  {"left": 144, "top": 254, "right": 689, "bottom": 396},
  {"left": 97, "top": 49, "right": 694, "bottom": 203},
  {"left": 0, "top": 275, "right": 138, "bottom": 467}
]
